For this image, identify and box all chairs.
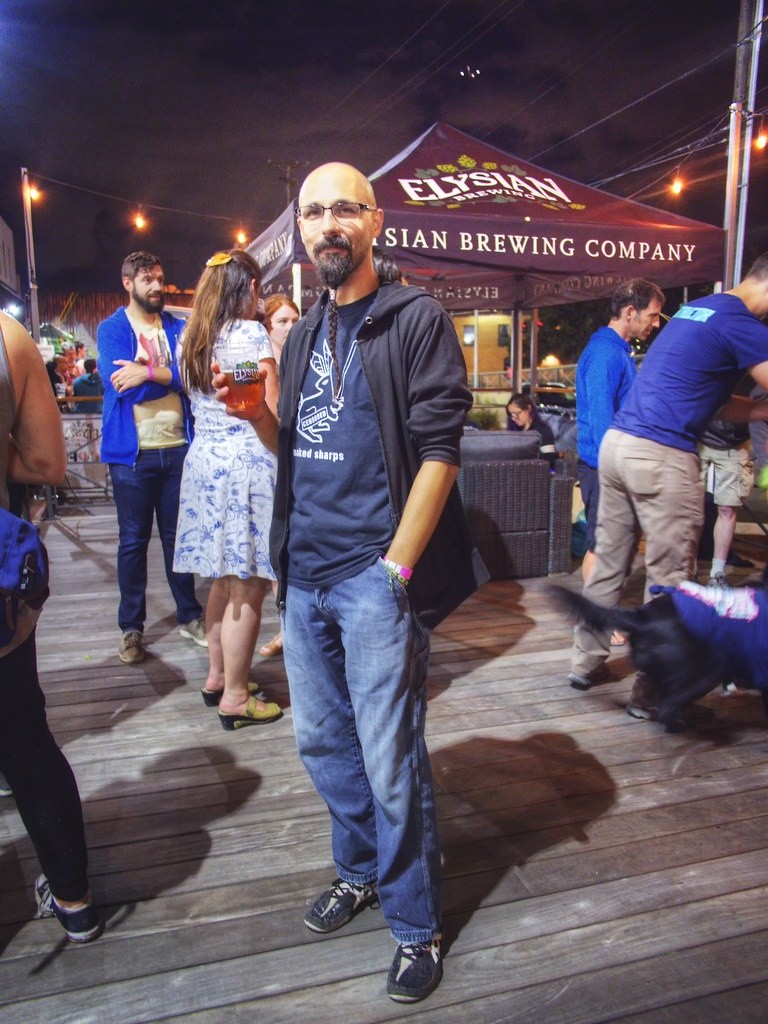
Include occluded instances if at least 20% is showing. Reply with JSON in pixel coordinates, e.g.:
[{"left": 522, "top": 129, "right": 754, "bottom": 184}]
[{"left": 454, "top": 430, "right": 575, "bottom": 578}]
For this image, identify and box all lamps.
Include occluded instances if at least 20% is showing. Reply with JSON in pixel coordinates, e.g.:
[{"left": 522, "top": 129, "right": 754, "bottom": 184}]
[{"left": 462, "top": 324, "right": 474, "bottom": 346}]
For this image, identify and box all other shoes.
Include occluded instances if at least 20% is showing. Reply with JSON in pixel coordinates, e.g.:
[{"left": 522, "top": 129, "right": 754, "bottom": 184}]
[
  {"left": 626, "top": 701, "right": 713, "bottom": 721},
  {"left": 568, "top": 664, "right": 609, "bottom": 686}
]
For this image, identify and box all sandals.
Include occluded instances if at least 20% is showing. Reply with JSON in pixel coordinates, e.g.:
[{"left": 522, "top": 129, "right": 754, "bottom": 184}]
[{"left": 259, "top": 632, "right": 283, "bottom": 657}]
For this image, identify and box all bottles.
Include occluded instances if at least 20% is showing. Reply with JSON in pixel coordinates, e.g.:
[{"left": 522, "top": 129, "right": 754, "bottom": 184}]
[{"left": 555, "top": 451, "right": 571, "bottom": 477}]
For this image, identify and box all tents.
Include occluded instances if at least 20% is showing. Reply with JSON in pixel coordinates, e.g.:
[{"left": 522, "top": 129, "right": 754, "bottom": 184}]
[{"left": 238, "top": 118, "right": 731, "bottom": 398}]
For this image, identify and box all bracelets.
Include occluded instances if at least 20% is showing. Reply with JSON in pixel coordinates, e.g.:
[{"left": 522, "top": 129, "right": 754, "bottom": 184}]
[{"left": 382, "top": 555, "right": 413, "bottom": 587}]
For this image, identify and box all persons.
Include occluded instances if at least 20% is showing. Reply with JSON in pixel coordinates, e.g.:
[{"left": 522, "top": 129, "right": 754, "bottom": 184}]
[
  {"left": 97, "top": 252, "right": 212, "bottom": 663},
  {"left": 0, "top": 303, "right": 103, "bottom": 943},
  {"left": 208, "top": 161, "right": 477, "bottom": 1005},
  {"left": 172, "top": 248, "right": 301, "bottom": 732},
  {"left": 566, "top": 257, "right": 768, "bottom": 723},
  {"left": 506, "top": 394, "right": 557, "bottom": 475}
]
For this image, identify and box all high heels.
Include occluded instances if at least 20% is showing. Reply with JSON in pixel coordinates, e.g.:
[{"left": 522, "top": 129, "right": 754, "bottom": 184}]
[
  {"left": 201, "top": 681, "right": 259, "bottom": 707},
  {"left": 218, "top": 695, "right": 283, "bottom": 731}
]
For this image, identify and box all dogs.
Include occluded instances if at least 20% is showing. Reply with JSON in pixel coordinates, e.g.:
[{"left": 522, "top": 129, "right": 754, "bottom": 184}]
[{"left": 538, "top": 578, "right": 768, "bottom": 735}]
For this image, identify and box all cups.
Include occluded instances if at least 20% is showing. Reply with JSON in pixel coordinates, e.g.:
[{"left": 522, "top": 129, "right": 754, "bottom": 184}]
[
  {"left": 56, "top": 383, "right": 66, "bottom": 398},
  {"left": 213, "top": 343, "right": 263, "bottom": 411}
]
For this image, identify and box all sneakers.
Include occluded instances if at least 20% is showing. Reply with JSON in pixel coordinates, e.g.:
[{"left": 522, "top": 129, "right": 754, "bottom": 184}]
[
  {"left": 34, "top": 872, "right": 103, "bottom": 944},
  {"left": 180, "top": 616, "right": 209, "bottom": 648},
  {"left": 387, "top": 939, "right": 443, "bottom": 1004},
  {"left": 118, "top": 631, "right": 146, "bottom": 663},
  {"left": 304, "top": 877, "right": 381, "bottom": 933},
  {"left": 708, "top": 572, "right": 732, "bottom": 588}
]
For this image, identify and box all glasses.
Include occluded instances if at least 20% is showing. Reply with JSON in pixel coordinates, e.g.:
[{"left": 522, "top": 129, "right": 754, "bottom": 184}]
[
  {"left": 508, "top": 410, "right": 525, "bottom": 419},
  {"left": 294, "top": 203, "right": 377, "bottom": 222}
]
